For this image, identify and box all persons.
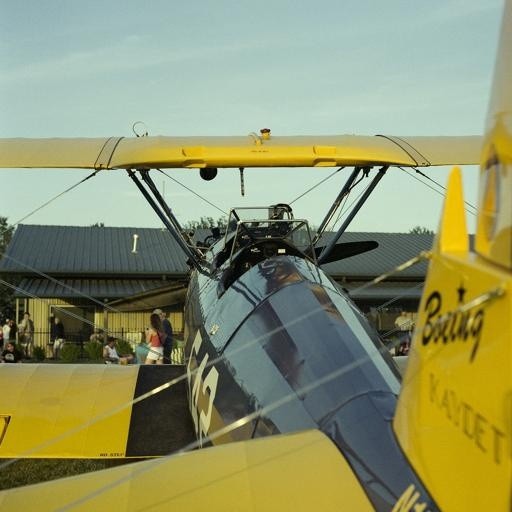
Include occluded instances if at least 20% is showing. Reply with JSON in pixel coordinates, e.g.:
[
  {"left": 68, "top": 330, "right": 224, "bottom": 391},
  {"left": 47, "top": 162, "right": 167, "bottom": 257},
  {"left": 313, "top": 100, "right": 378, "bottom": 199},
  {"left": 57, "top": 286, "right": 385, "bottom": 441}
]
[
  {"left": 19, "top": 312, "right": 34, "bottom": 359},
  {"left": 153, "top": 309, "right": 172, "bottom": 364},
  {"left": 2, "top": 318, "right": 12, "bottom": 352},
  {"left": 0, "top": 341, "right": 24, "bottom": 362},
  {"left": 142, "top": 313, "right": 164, "bottom": 365},
  {"left": 48, "top": 313, "right": 67, "bottom": 359},
  {"left": 103, "top": 337, "right": 137, "bottom": 363},
  {"left": 394, "top": 308, "right": 415, "bottom": 331}
]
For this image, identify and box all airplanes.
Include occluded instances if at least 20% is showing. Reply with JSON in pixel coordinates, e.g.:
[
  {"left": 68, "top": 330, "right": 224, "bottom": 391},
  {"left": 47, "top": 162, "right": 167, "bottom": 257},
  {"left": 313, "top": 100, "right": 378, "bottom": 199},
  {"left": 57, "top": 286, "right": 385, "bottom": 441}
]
[{"left": 0, "top": 0, "right": 511, "bottom": 511}]
